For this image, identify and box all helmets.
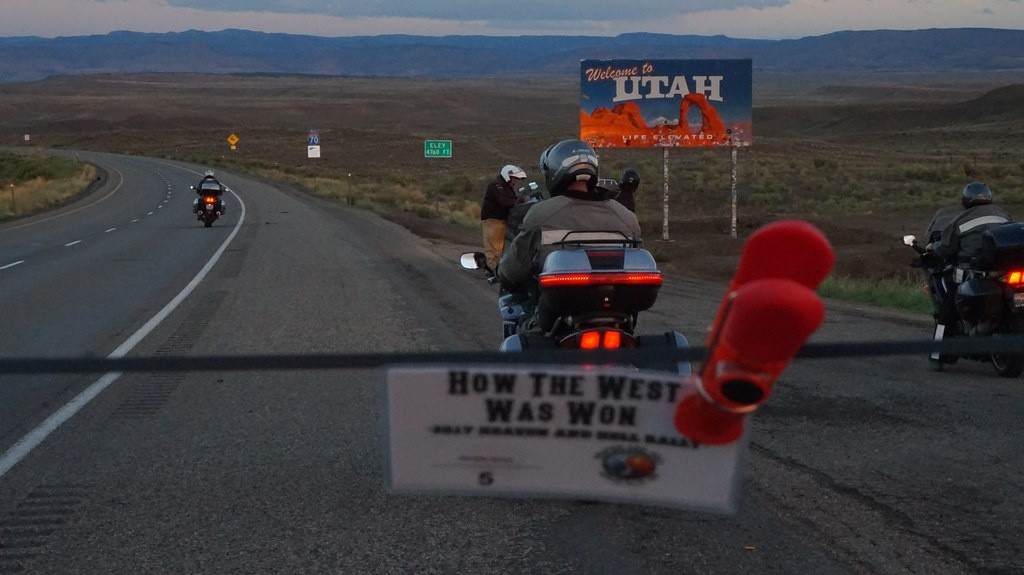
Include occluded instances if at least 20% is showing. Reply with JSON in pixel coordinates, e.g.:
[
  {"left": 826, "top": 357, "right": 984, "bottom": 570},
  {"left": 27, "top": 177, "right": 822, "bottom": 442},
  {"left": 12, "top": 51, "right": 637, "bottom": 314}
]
[
  {"left": 538, "top": 139, "right": 600, "bottom": 195},
  {"left": 205, "top": 170, "right": 215, "bottom": 178},
  {"left": 962, "top": 182, "right": 992, "bottom": 209},
  {"left": 500, "top": 165, "right": 528, "bottom": 183}
]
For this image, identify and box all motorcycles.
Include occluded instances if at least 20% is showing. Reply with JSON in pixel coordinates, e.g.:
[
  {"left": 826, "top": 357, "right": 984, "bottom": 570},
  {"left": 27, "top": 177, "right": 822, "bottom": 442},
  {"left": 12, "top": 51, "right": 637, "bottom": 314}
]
[
  {"left": 505, "top": 181, "right": 543, "bottom": 242},
  {"left": 459, "top": 245, "right": 692, "bottom": 354},
  {"left": 903, "top": 221, "right": 1024, "bottom": 380},
  {"left": 190, "top": 185, "right": 229, "bottom": 229}
]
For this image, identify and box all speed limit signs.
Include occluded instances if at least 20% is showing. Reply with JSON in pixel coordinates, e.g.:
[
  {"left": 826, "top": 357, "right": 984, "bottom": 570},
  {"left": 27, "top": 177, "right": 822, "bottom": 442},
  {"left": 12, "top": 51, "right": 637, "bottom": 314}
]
[{"left": 308, "top": 129, "right": 320, "bottom": 158}]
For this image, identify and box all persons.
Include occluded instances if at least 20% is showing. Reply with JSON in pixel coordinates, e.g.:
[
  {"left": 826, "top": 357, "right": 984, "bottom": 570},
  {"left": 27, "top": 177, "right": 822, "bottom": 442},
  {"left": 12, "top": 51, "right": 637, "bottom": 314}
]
[
  {"left": 497, "top": 138, "right": 643, "bottom": 351},
  {"left": 614, "top": 167, "right": 640, "bottom": 213},
  {"left": 925, "top": 182, "right": 1013, "bottom": 371},
  {"left": 480, "top": 164, "right": 527, "bottom": 276},
  {"left": 193, "top": 169, "right": 226, "bottom": 215}
]
